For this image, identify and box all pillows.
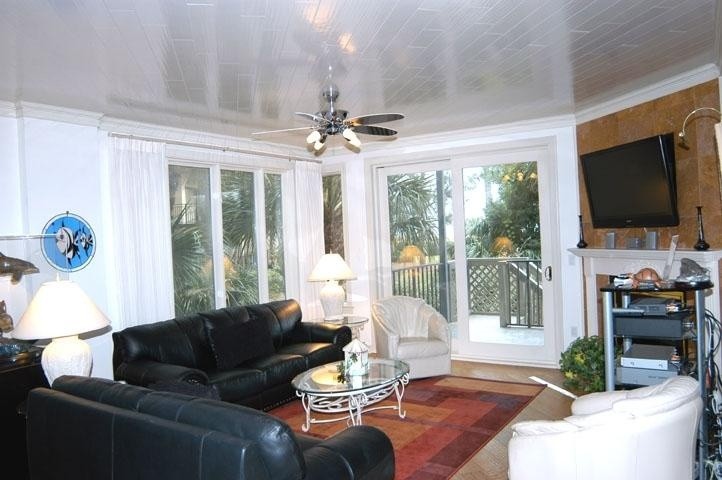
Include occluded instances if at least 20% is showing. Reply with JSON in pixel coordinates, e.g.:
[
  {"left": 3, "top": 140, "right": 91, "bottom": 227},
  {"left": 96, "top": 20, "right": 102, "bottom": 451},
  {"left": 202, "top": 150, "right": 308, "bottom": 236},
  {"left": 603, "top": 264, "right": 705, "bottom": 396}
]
[
  {"left": 148, "top": 377, "right": 222, "bottom": 402},
  {"left": 211, "top": 318, "right": 277, "bottom": 372}
]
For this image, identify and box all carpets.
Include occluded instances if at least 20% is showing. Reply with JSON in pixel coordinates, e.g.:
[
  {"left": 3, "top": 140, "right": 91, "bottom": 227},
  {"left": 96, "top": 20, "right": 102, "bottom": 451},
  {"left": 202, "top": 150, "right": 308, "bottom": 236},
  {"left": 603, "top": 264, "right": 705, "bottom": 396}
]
[{"left": 266, "top": 376, "right": 546, "bottom": 480}]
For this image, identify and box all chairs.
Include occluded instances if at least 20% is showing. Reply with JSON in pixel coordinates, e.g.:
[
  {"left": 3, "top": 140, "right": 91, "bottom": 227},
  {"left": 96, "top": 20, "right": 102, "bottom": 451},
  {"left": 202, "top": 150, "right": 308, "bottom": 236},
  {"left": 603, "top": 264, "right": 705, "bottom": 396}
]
[
  {"left": 373, "top": 293, "right": 451, "bottom": 380},
  {"left": 507, "top": 376, "right": 706, "bottom": 480}
]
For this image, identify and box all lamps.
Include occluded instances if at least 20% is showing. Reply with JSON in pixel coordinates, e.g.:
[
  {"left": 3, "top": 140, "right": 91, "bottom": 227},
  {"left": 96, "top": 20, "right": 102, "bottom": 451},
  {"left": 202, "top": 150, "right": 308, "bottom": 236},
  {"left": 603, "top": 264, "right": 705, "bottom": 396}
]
[
  {"left": 306, "top": 127, "right": 362, "bottom": 149},
  {"left": 17, "top": 280, "right": 114, "bottom": 386},
  {"left": 308, "top": 253, "right": 359, "bottom": 322},
  {"left": 678, "top": 107, "right": 722, "bottom": 144}
]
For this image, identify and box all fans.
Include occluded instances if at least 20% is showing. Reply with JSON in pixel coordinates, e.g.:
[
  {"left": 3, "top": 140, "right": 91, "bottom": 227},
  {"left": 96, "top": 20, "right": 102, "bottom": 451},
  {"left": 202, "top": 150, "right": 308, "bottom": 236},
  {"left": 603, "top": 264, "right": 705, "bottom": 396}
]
[{"left": 252, "top": 83, "right": 405, "bottom": 137}]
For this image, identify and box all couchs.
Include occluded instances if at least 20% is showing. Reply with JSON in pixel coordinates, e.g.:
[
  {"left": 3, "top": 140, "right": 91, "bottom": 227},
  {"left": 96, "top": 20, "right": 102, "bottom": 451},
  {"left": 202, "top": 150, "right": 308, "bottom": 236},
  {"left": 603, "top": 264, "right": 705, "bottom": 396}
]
[
  {"left": 112, "top": 300, "right": 353, "bottom": 411},
  {"left": 24, "top": 376, "right": 395, "bottom": 480}
]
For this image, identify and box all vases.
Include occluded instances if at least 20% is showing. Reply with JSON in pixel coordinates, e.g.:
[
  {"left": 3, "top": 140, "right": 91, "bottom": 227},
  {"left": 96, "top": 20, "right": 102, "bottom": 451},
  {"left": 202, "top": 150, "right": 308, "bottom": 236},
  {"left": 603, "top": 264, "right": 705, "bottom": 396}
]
[
  {"left": 694, "top": 206, "right": 710, "bottom": 250},
  {"left": 577, "top": 215, "right": 588, "bottom": 248}
]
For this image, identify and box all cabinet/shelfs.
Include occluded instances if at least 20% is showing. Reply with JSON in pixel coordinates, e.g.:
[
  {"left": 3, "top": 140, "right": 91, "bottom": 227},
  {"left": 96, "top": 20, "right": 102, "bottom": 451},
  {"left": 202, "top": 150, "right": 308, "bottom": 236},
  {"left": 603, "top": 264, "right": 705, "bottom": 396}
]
[
  {"left": 601, "top": 283, "right": 715, "bottom": 393},
  {"left": 0, "top": 334, "right": 50, "bottom": 480}
]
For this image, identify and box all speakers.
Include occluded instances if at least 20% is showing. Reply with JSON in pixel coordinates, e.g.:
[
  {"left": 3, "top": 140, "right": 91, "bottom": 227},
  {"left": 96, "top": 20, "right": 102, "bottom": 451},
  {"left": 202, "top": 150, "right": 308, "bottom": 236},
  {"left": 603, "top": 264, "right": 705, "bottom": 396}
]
[
  {"left": 606, "top": 232, "right": 615, "bottom": 248},
  {"left": 646, "top": 231, "right": 657, "bottom": 249}
]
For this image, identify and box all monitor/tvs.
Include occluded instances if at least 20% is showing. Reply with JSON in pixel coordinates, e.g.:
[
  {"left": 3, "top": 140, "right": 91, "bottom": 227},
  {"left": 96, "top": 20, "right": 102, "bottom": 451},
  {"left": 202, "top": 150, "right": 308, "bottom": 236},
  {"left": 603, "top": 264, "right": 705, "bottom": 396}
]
[{"left": 580, "top": 135, "right": 679, "bottom": 228}]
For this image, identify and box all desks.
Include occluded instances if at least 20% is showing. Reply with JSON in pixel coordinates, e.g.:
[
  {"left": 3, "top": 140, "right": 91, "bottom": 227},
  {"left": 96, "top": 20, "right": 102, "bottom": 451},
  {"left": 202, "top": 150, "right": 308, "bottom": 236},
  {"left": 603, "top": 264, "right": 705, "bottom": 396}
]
[{"left": 316, "top": 318, "right": 368, "bottom": 341}]
